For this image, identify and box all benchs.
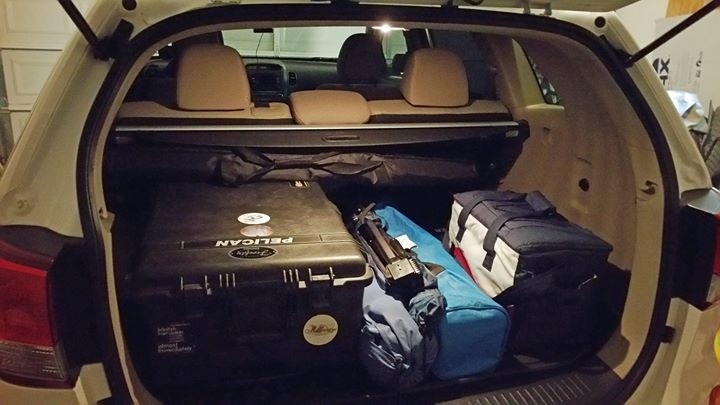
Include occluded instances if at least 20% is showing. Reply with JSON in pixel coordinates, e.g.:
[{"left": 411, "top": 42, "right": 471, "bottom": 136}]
[{"left": 101, "top": 33, "right": 530, "bottom": 238}]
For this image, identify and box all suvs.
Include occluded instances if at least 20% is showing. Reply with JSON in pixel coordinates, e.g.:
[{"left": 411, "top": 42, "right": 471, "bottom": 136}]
[{"left": 0, "top": 0, "right": 719, "bottom": 404}]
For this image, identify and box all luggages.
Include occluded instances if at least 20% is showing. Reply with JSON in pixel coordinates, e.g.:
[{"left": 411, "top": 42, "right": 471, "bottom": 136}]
[{"left": 130, "top": 180, "right": 373, "bottom": 382}]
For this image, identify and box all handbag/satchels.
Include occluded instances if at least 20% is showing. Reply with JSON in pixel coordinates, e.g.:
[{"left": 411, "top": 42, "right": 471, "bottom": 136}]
[
  {"left": 373, "top": 203, "right": 512, "bottom": 380},
  {"left": 441, "top": 188, "right": 614, "bottom": 361}
]
